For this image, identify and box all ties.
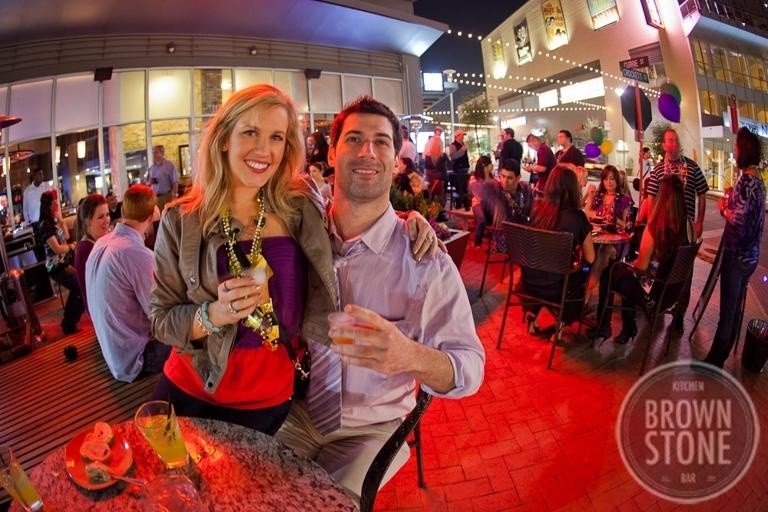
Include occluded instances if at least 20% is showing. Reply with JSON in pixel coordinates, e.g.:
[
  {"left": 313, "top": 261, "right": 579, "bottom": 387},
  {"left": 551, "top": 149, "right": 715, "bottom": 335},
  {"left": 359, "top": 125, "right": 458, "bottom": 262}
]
[{"left": 307, "top": 235, "right": 369, "bottom": 437}]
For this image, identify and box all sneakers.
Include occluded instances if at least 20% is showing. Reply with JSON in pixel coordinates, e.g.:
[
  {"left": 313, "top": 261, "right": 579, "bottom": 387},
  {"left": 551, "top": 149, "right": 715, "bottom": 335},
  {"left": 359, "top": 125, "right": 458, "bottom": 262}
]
[
  {"left": 666, "top": 314, "right": 683, "bottom": 338},
  {"left": 475, "top": 234, "right": 481, "bottom": 247},
  {"left": 64, "top": 326, "right": 83, "bottom": 335}
]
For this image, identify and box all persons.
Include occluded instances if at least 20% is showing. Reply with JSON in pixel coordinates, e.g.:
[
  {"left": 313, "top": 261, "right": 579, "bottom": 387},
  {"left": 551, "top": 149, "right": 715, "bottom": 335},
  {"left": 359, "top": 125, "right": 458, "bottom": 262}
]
[{"left": 2, "top": 84, "right": 767, "bottom": 510}]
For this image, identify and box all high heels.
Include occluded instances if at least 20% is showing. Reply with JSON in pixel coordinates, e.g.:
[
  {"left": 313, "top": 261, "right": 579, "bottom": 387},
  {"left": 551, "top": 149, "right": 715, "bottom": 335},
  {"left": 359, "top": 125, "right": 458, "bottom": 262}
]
[
  {"left": 550, "top": 320, "right": 565, "bottom": 346},
  {"left": 526, "top": 311, "right": 544, "bottom": 336}
]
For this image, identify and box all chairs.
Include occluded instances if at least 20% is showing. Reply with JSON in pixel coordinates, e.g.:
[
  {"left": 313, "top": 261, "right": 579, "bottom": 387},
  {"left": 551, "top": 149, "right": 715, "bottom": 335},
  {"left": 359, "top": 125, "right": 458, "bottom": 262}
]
[{"left": 360, "top": 386, "right": 434, "bottom": 512}]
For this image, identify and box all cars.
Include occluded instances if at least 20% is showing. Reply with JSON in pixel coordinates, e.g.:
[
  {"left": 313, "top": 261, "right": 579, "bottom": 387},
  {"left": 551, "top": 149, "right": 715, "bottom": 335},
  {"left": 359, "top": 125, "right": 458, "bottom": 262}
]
[{"left": 584, "top": 157, "right": 608, "bottom": 178}]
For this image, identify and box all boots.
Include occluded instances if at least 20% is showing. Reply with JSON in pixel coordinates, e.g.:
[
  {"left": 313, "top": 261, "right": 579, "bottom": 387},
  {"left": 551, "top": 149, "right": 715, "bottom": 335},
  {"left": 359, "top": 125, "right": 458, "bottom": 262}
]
[
  {"left": 614, "top": 294, "right": 638, "bottom": 343},
  {"left": 586, "top": 287, "right": 612, "bottom": 346}
]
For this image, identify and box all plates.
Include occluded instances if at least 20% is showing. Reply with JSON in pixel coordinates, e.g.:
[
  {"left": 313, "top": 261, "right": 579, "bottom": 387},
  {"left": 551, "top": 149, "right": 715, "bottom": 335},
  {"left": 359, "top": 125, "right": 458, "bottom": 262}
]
[
  {"left": 61, "top": 426, "right": 134, "bottom": 491},
  {"left": 589, "top": 222, "right": 608, "bottom": 226}
]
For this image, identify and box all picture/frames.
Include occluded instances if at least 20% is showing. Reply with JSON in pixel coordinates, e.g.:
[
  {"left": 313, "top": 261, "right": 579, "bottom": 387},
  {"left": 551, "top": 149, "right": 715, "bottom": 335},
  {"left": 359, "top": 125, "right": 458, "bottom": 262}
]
[{"left": 179, "top": 145, "right": 190, "bottom": 176}]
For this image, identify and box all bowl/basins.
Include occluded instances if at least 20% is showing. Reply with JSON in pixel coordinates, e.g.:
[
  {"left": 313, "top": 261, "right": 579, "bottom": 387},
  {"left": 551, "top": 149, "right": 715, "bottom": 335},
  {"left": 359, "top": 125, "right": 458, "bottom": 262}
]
[{"left": 7, "top": 250, "right": 38, "bottom": 273}]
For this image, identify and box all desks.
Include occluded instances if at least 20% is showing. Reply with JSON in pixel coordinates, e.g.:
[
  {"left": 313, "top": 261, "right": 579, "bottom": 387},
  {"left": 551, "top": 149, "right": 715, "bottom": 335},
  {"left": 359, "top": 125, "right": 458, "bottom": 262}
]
[{"left": 9, "top": 416, "right": 358, "bottom": 511}]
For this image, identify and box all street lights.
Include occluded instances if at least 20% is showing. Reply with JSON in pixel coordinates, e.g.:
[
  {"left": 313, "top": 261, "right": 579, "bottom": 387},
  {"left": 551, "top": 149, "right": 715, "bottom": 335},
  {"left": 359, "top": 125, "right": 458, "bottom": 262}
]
[
  {"left": 439, "top": 67, "right": 457, "bottom": 145},
  {"left": 411, "top": 123, "right": 422, "bottom": 165}
]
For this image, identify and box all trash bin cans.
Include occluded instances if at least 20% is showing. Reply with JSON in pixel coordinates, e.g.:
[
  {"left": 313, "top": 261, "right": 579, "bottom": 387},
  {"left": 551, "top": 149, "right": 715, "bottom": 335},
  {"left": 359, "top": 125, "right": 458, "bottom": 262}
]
[{"left": 741, "top": 319, "right": 768, "bottom": 373}]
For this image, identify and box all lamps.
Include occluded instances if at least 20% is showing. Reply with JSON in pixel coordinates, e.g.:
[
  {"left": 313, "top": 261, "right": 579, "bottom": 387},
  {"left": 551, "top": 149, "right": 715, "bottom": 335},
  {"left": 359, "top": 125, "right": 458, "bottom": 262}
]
[
  {"left": 249, "top": 47, "right": 256, "bottom": 56},
  {"left": 77, "top": 133, "right": 86, "bottom": 158},
  {"left": 166, "top": 41, "right": 176, "bottom": 53},
  {"left": 56, "top": 137, "right": 61, "bottom": 163}
]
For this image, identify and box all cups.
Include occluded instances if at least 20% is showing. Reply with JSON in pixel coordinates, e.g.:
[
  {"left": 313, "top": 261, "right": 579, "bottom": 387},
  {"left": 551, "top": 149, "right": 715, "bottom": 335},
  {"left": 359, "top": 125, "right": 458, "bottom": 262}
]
[
  {"left": 134, "top": 398, "right": 192, "bottom": 472},
  {"left": 241, "top": 267, "right": 271, "bottom": 307},
  {"left": 327, "top": 310, "right": 359, "bottom": 356},
  {"left": 1, "top": 443, "right": 47, "bottom": 511},
  {"left": 591, "top": 216, "right": 604, "bottom": 224}
]
